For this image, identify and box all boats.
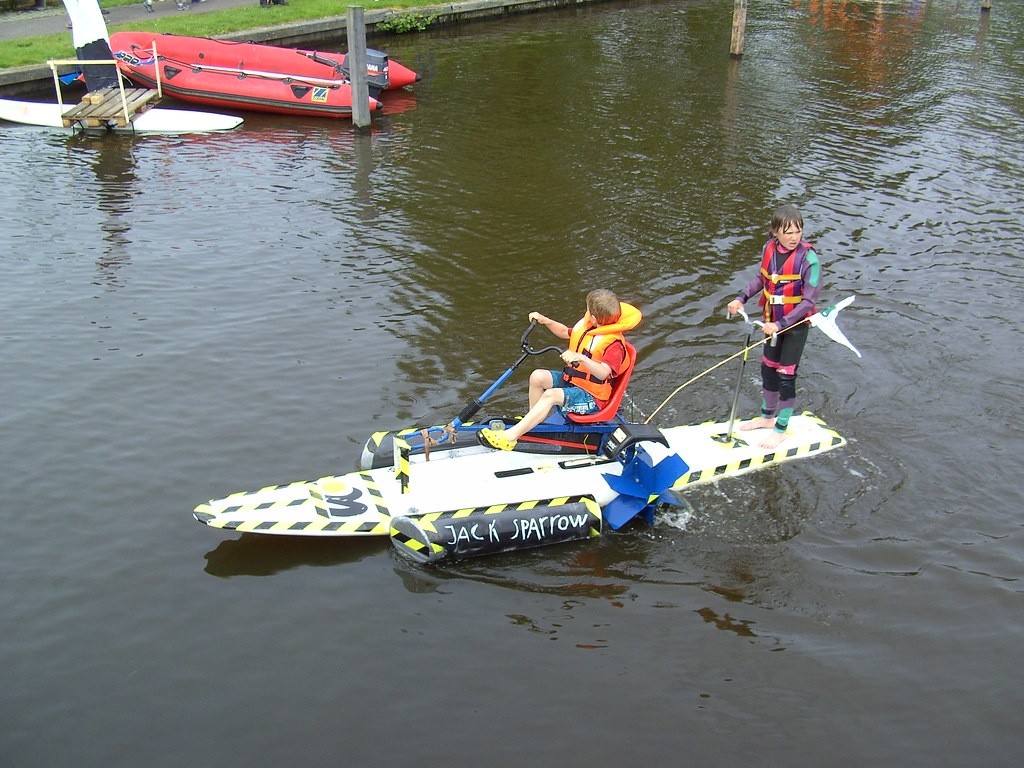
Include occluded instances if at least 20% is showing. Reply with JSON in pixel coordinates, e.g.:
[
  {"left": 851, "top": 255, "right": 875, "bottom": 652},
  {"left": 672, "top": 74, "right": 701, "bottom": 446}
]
[
  {"left": 191, "top": 313, "right": 848, "bottom": 566},
  {"left": 108, "top": 31, "right": 422, "bottom": 119}
]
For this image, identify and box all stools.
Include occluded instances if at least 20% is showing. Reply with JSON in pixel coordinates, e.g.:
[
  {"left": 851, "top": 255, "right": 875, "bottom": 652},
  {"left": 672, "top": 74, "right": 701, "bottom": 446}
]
[{"left": 62, "top": 87, "right": 158, "bottom": 127}]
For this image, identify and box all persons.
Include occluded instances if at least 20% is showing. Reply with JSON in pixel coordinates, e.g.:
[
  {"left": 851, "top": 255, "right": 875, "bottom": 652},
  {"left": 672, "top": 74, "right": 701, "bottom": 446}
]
[
  {"left": 727, "top": 205, "right": 822, "bottom": 451},
  {"left": 477, "top": 289, "right": 644, "bottom": 451}
]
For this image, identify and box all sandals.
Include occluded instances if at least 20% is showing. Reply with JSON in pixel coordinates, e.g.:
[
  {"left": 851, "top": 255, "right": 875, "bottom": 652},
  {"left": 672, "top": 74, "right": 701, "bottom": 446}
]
[
  {"left": 514, "top": 416, "right": 525, "bottom": 421},
  {"left": 481, "top": 427, "right": 517, "bottom": 452}
]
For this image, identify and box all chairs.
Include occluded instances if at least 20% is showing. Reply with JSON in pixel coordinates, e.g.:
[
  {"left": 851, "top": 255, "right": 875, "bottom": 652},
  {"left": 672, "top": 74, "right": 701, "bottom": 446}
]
[{"left": 561, "top": 341, "right": 637, "bottom": 422}]
